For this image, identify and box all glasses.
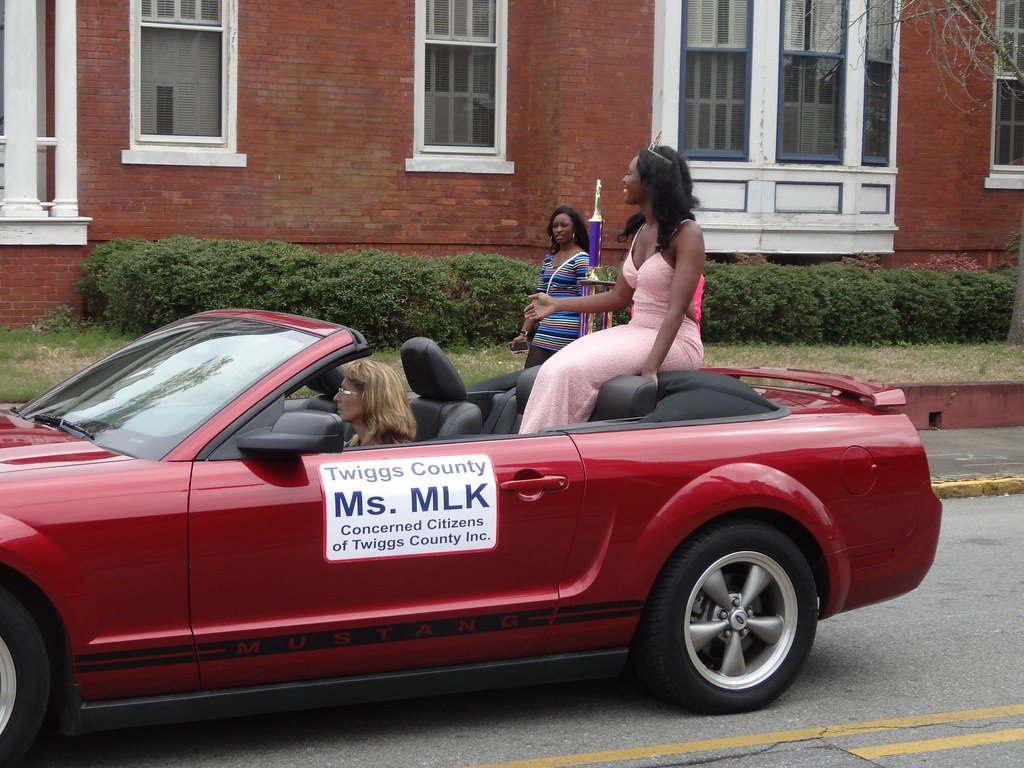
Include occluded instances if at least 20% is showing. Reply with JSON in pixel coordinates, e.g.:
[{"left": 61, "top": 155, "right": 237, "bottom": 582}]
[{"left": 339, "top": 388, "right": 361, "bottom": 395}]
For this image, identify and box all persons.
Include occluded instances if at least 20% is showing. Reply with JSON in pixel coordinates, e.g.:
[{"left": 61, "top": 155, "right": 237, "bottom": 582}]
[
  {"left": 512, "top": 130, "right": 706, "bottom": 434},
  {"left": 333, "top": 360, "right": 417, "bottom": 448}
]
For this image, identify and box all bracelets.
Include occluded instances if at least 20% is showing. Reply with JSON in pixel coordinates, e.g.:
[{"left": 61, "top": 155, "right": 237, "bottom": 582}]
[{"left": 520, "top": 330, "right": 530, "bottom": 337}]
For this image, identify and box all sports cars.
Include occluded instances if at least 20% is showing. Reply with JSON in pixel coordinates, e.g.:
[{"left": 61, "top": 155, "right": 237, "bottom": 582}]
[{"left": 1, "top": 307, "right": 943, "bottom": 768}]
